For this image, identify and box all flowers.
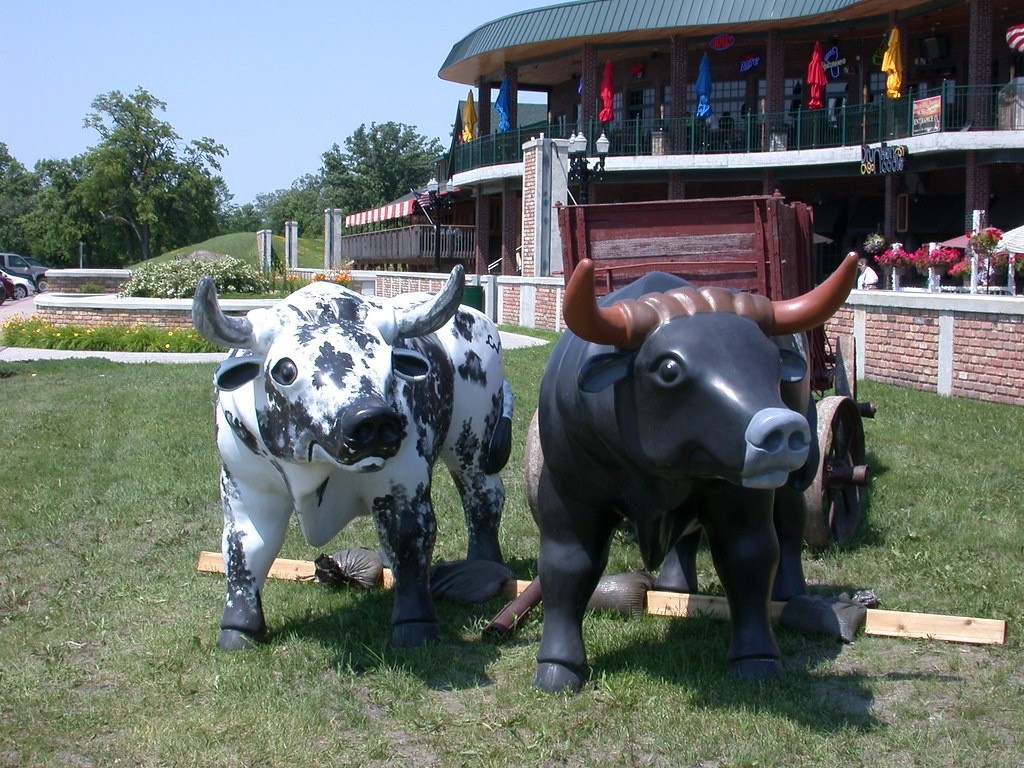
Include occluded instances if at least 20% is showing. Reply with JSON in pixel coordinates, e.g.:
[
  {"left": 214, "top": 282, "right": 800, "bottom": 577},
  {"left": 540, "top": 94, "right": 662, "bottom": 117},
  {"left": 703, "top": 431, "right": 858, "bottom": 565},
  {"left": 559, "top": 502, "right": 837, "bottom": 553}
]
[
  {"left": 874, "top": 250, "right": 914, "bottom": 276},
  {"left": 907, "top": 243, "right": 961, "bottom": 276},
  {"left": 949, "top": 256, "right": 985, "bottom": 283},
  {"left": 966, "top": 226, "right": 1006, "bottom": 257},
  {"left": 863, "top": 233, "right": 887, "bottom": 254},
  {"left": 1008, "top": 253, "right": 1024, "bottom": 272},
  {"left": 989, "top": 253, "right": 1008, "bottom": 276}
]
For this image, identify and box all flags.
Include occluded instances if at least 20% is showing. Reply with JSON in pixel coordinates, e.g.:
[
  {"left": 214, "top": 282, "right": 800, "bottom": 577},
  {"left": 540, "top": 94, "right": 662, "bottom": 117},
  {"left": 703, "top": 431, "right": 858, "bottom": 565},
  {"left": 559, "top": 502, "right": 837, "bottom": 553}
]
[{"left": 414, "top": 192, "right": 430, "bottom": 206}]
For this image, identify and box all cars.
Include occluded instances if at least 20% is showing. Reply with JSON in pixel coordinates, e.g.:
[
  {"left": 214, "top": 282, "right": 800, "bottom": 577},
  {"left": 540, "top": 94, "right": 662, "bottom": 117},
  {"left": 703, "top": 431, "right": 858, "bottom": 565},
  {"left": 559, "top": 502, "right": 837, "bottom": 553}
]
[
  {"left": 0, "top": 253, "right": 52, "bottom": 292},
  {"left": 0, "top": 265, "right": 37, "bottom": 304}
]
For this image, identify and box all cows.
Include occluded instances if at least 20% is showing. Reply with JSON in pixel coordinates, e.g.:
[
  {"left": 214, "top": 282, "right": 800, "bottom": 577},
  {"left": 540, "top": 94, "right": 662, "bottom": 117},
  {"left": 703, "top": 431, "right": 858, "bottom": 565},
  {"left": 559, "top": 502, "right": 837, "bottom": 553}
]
[
  {"left": 191, "top": 276, "right": 516, "bottom": 650},
  {"left": 522, "top": 251, "right": 861, "bottom": 694}
]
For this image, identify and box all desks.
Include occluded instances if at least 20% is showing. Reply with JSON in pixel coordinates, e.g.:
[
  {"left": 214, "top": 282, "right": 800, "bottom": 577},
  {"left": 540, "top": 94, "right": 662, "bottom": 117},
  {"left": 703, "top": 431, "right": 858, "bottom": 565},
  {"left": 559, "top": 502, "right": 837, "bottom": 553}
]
[{"left": 711, "top": 128, "right": 745, "bottom": 153}]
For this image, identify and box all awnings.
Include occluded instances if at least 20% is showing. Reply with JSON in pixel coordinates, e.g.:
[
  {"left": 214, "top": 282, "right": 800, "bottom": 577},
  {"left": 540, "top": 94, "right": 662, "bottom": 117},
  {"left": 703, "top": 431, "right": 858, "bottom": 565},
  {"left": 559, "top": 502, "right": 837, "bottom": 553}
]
[{"left": 346, "top": 186, "right": 459, "bottom": 229}]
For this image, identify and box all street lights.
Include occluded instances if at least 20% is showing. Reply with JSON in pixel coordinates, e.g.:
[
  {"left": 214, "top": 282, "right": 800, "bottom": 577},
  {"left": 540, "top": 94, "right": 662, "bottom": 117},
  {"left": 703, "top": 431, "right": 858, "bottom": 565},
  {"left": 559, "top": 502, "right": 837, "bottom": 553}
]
[
  {"left": 567, "top": 131, "right": 609, "bottom": 204},
  {"left": 427, "top": 178, "right": 454, "bottom": 272}
]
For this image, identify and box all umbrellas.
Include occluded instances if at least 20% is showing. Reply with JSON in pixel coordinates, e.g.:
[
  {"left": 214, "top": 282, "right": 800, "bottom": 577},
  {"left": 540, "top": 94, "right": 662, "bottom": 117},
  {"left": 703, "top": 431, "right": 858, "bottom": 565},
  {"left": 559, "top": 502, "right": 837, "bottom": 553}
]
[
  {"left": 814, "top": 233, "right": 833, "bottom": 244},
  {"left": 599, "top": 60, "right": 614, "bottom": 122},
  {"left": 941, "top": 227, "right": 1006, "bottom": 295},
  {"left": 695, "top": 53, "right": 713, "bottom": 118},
  {"left": 494, "top": 78, "right": 512, "bottom": 132},
  {"left": 881, "top": 28, "right": 902, "bottom": 100},
  {"left": 988, "top": 226, "right": 1024, "bottom": 254},
  {"left": 463, "top": 89, "right": 477, "bottom": 142},
  {"left": 807, "top": 42, "right": 827, "bottom": 110}
]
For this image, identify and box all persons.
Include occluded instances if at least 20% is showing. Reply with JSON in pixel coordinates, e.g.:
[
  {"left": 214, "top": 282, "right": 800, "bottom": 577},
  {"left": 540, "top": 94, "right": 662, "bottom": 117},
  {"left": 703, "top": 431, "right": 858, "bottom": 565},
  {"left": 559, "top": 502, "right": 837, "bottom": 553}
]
[{"left": 857, "top": 258, "right": 878, "bottom": 290}]
